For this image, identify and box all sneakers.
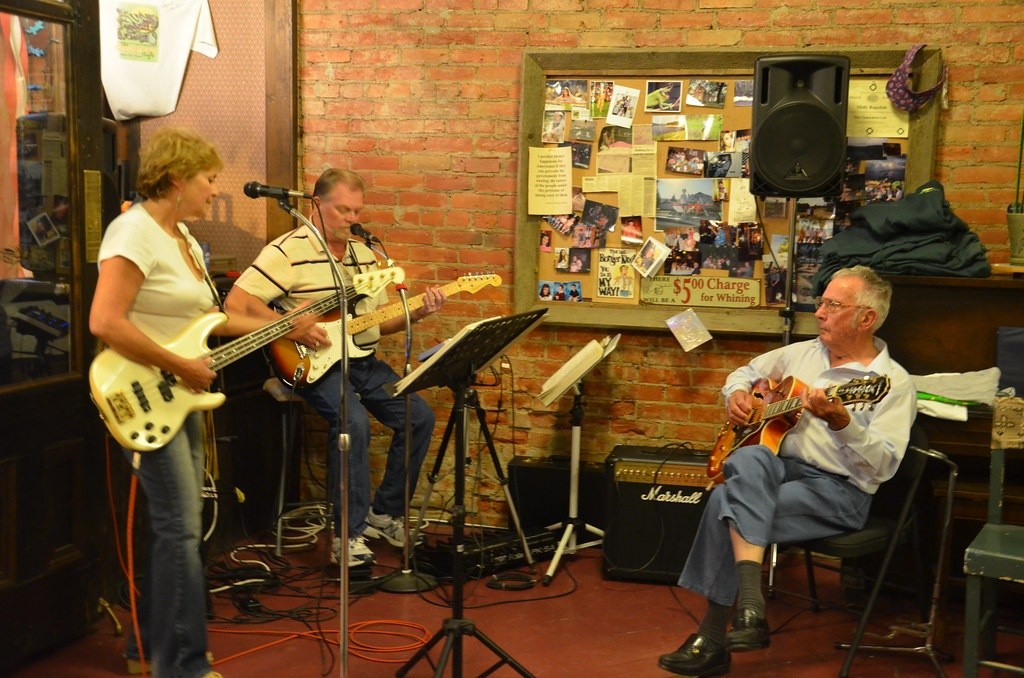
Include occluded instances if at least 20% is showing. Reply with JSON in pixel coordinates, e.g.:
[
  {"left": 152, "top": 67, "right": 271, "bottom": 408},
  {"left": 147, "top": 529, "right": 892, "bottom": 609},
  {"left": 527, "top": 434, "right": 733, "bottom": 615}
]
[
  {"left": 360, "top": 506, "right": 426, "bottom": 547},
  {"left": 330, "top": 534, "right": 377, "bottom": 569}
]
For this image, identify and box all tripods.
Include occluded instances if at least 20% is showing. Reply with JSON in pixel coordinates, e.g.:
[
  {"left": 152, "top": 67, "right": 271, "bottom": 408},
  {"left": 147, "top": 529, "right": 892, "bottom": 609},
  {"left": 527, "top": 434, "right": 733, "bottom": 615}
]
[
  {"left": 393, "top": 308, "right": 549, "bottom": 678},
  {"left": 833, "top": 446, "right": 956, "bottom": 678},
  {"left": 542, "top": 333, "right": 621, "bottom": 587}
]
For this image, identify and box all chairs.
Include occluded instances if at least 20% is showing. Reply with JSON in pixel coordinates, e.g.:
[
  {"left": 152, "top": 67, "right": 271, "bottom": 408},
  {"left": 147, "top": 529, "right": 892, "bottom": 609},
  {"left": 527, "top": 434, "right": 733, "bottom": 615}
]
[
  {"left": 767, "top": 417, "right": 929, "bottom": 625},
  {"left": 963, "top": 393, "right": 1024, "bottom": 678}
]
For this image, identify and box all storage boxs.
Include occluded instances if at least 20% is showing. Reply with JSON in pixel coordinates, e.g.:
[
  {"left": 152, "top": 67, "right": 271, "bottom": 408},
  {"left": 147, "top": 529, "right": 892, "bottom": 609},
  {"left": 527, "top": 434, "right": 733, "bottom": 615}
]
[{"left": 507, "top": 453, "right": 605, "bottom": 541}]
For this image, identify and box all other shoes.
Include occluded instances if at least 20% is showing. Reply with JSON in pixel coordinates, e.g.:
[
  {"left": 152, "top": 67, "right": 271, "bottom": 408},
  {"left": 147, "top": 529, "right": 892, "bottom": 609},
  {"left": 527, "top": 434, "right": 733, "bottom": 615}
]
[
  {"left": 204, "top": 670, "right": 223, "bottom": 678},
  {"left": 128, "top": 650, "right": 214, "bottom": 674}
]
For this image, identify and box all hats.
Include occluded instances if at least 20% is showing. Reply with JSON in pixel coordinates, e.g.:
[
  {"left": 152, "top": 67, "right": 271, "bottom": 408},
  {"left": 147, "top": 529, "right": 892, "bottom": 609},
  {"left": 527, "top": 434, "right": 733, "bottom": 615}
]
[{"left": 885, "top": 44, "right": 945, "bottom": 111}]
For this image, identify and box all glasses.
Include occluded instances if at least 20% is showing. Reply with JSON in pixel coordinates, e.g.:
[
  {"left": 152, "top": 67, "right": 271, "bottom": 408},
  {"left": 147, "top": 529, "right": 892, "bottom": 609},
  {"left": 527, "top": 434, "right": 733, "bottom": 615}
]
[{"left": 815, "top": 296, "right": 871, "bottom": 312}]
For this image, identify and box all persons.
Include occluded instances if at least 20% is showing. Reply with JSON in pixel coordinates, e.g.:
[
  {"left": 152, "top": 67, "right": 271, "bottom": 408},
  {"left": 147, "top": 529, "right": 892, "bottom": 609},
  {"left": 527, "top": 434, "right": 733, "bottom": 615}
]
[
  {"left": 554, "top": 87, "right": 575, "bottom": 105},
  {"left": 714, "top": 132, "right": 751, "bottom": 178},
  {"left": 657, "top": 266, "right": 918, "bottom": 677},
  {"left": 547, "top": 112, "right": 615, "bottom": 167},
  {"left": 842, "top": 163, "right": 902, "bottom": 205},
  {"left": 538, "top": 189, "right": 607, "bottom": 302},
  {"left": 667, "top": 150, "right": 703, "bottom": 174},
  {"left": 627, "top": 181, "right": 788, "bottom": 305},
  {"left": 224, "top": 171, "right": 446, "bottom": 568},
  {"left": 697, "top": 81, "right": 754, "bottom": 104},
  {"left": 796, "top": 209, "right": 826, "bottom": 274},
  {"left": 595, "top": 84, "right": 632, "bottom": 116},
  {"left": 33, "top": 220, "right": 54, "bottom": 243},
  {"left": 88, "top": 128, "right": 314, "bottom": 678}
]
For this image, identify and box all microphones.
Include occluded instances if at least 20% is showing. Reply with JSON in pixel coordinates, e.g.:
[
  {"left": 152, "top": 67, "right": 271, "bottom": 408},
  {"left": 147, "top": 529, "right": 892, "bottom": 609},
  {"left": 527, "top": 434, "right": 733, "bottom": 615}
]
[
  {"left": 349, "top": 223, "right": 379, "bottom": 242},
  {"left": 242, "top": 180, "right": 313, "bottom": 200}
]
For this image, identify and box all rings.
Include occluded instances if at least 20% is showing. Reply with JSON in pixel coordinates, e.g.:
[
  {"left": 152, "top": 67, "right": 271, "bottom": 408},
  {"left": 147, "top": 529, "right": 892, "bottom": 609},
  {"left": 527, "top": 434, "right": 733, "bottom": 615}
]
[
  {"left": 431, "top": 302, "right": 435, "bottom": 306},
  {"left": 316, "top": 342, "right": 319, "bottom": 347}
]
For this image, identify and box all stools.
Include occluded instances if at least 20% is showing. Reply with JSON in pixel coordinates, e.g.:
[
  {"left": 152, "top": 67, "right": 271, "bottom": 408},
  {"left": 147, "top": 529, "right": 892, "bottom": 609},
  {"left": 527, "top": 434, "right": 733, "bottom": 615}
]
[{"left": 261, "top": 372, "right": 362, "bottom": 558}]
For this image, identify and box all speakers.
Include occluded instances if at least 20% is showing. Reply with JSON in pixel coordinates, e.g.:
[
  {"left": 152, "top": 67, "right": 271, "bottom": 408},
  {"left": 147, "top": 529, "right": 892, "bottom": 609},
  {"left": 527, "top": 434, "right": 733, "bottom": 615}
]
[
  {"left": 750, "top": 53, "right": 851, "bottom": 202},
  {"left": 601, "top": 445, "right": 714, "bottom": 586}
]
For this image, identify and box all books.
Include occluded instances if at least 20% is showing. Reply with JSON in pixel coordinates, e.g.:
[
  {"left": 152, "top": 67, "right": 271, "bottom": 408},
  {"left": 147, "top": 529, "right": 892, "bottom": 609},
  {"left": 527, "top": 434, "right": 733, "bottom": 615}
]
[
  {"left": 395, "top": 316, "right": 501, "bottom": 394},
  {"left": 538, "top": 336, "right": 610, "bottom": 408}
]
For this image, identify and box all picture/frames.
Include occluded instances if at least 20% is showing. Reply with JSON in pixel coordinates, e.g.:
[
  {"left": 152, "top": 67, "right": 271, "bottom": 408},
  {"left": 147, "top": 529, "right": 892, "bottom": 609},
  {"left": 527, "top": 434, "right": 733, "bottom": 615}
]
[{"left": 510, "top": 42, "right": 945, "bottom": 337}]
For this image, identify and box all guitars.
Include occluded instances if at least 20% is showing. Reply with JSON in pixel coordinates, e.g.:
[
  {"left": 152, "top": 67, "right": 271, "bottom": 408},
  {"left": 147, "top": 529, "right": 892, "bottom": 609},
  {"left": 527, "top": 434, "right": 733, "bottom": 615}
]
[
  {"left": 265, "top": 269, "right": 503, "bottom": 394},
  {"left": 706, "top": 373, "right": 892, "bottom": 484},
  {"left": 86, "top": 258, "right": 407, "bottom": 455}
]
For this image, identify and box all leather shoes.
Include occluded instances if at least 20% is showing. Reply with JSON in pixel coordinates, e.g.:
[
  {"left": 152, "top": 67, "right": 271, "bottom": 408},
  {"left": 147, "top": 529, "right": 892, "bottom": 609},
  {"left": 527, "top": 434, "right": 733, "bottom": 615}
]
[
  {"left": 658, "top": 632, "right": 730, "bottom": 677},
  {"left": 726, "top": 616, "right": 770, "bottom": 650}
]
